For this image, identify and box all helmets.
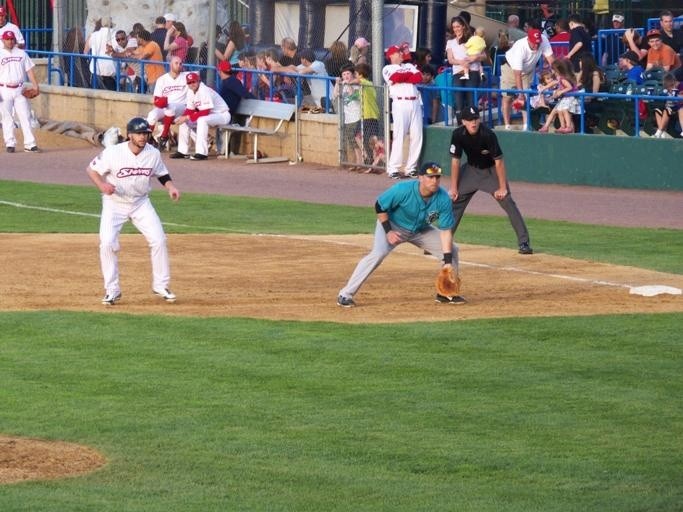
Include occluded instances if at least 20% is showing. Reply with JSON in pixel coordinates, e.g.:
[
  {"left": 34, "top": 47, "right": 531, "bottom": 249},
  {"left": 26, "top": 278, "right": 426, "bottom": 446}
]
[{"left": 127, "top": 117, "right": 153, "bottom": 132}]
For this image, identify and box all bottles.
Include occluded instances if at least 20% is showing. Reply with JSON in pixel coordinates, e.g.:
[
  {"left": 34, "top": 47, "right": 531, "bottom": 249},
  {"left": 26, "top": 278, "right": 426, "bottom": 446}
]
[
  {"left": 553, "top": 85, "right": 558, "bottom": 101},
  {"left": 625, "top": 84, "right": 632, "bottom": 101},
  {"left": 344, "top": 92, "right": 360, "bottom": 104}
]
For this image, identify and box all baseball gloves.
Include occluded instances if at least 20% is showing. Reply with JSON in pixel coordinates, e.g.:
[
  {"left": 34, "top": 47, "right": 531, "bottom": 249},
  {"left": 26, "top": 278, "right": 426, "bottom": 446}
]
[
  {"left": 22, "top": 88, "right": 38, "bottom": 99},
  {"left": 436, "top": 264, "right": 462, "bottom": 297}
]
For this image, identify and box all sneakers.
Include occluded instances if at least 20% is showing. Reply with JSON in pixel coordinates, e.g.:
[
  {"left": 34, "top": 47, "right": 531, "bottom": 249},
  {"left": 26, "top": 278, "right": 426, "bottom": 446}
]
[
  {"left": 435, "top": 292, "right": 467, "bottom": 305},
  {"left": 23, "top": 146, "right": 40, "bottom": 153},
  {"left": 390, "top": 172, "right": 402, "bottom": 180},
  {"left": 151, "top": 286, "right": 177, "bottom": 301},
  {"left": 157, "top": 136, "right": 209, "bottom": 160},
  {"left": 457, "top": 73, "right": 470, "bottom": 80},
  {"left": 6, "top": 147, "right": 15, "bottom": 153},
  {"left": 480, "top": 72, "right": 486, "bottom": 83},
  {"left": 337, "top": 295, "right": 356, "bottom": 308},
  {"left": 101, "top": 291, "right": 121, "bottom": 305},
  {"left": 517, "top": 241, "right": 533, "bottom": 254},
  {"left": 403, "top": 171, "right": 418, "bottom": 179},
  {"left": 504, "top": 123, "right": 574, "bottom": 134}
]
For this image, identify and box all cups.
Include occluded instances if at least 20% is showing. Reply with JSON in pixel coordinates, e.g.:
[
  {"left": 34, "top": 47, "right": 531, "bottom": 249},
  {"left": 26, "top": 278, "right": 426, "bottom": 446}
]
[{"left": 671, "top": 89, "right": 678, "bottom": 97}]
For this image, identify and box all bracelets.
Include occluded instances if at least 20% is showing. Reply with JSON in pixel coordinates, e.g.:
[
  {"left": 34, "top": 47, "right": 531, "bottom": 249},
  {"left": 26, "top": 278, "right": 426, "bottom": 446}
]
[
  {"left": 443, "top": 253, "right": 453, "bottom": 264},
  {"left": 381, "top": 220, "right": 391, "bottom": 234}
]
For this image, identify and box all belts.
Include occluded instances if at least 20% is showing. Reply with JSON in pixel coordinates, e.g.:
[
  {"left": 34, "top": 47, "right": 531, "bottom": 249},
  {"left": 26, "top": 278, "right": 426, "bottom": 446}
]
[
  {"left": 0, "top": 82, "right": 23, "bottom": 88},
  {"left": 391, "top": 96, "right": 418, "bottom": 101}
]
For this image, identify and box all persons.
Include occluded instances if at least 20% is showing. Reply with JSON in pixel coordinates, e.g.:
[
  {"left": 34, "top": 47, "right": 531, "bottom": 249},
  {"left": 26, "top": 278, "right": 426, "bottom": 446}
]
[
  {"left": 335, "top": 161, "right": 467, "bottom": 308},
  {"left": 446, "top": 103, "right": 534, "bottom": 254},
  {"left": 0, "top": 6, "right": 26, "bottom": 49},
  {"left": 85, "top": 117, "right": 181, "bottom": 305},
  {"left": 81, "top": 10, "right": 683, "bottom": 180},
  {"left": 0, "top": 30, "right": 43, "bottom": 153}
]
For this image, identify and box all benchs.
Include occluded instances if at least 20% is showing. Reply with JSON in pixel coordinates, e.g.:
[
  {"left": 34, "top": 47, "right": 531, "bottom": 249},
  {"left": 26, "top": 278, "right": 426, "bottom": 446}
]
[{"left": 166, "top": 99, "right": 295, "bottom": 164}]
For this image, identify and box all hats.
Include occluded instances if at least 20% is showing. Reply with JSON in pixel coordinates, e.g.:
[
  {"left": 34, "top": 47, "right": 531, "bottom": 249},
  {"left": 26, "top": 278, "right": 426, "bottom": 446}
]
[
  {"left": 186, "top": 73, "right": 199, "bottom": 84},
  {"left": 354, "top": 38, "right": 370, "bottom": 49},
  {"left": 0, "top": 8, "right": 8, "bottom": 16},
  {"left": 526, "top": 29, "right": 543, "bottom": 44},
  {"left": 645, "top": 29, "right": 662, "bottom": 38},
  {"left": 164, "top": 13, "right": 176, "bottom": 21},
  {"left": 419, "top": 162, "right": 444, "bottom": 177},
  {"left": 385, "top": 45, "right": 405, "bottom": 61},
  {"left": 611, "top": 12, "right": 626, "bottom": 23},
  {"left": 2, "top": 31, "right": 15, "bottom": 39},
  {"left": 461, "top": 105, "right": 479, "bottom": 121},
  {"left": 216, "top": 61, "right": 231, "bottom": 71}
]
